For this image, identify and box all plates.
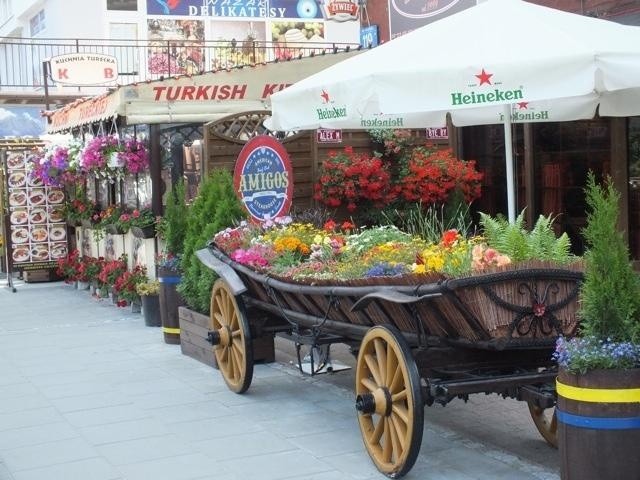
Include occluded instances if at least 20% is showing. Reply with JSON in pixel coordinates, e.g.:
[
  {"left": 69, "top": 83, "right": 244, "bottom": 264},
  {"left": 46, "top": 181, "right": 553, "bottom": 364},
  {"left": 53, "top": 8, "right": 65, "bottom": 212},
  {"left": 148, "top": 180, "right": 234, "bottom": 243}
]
[{"left": 6, "top": 153, "right": 68, "bottom": 262}]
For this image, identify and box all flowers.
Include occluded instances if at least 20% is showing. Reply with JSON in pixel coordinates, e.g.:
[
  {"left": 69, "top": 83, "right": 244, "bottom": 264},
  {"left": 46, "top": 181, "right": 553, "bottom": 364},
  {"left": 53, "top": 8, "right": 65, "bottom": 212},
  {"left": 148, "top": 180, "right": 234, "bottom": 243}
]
[
  {"left": 56, "top": 249, "right": 148, "bottom": 307},
  {"left": 119, "top": 202, "right": 155, "bottom": 226},
  {"left": 29, "top": 133, "right": 150, "bottom": 189},
  {"left": 551, "top": 329, "right": 640, "bottom": 378},
  {"left": 72, "top": 197, "right": 100, "bottom": 223},
  {"left": 100, "top": 204, "right": 120, "bottom": 225}
]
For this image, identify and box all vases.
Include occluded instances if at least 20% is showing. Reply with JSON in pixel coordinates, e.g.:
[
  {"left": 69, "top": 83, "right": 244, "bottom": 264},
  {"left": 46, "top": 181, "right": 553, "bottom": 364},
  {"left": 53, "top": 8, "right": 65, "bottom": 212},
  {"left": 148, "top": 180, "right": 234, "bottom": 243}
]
[
  {"left": 555, "top": 367, "right": 640, "bottom": 480},
  {"left": 130, "top": 300, "right": 141, "bottom": 313},
  {"left": 81, "top": 218, "right": 100, "bottom": 229},
  {"left": 130, "top": 223, "right": 156, "bottom": 238},
  {"left": 107, "top": 223, "right": 122, "bottom": 235}
]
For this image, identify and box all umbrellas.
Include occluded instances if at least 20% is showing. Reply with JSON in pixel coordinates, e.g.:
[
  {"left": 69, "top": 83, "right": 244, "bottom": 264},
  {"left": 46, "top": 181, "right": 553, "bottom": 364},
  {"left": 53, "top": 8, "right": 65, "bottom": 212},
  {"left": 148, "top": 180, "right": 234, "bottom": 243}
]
[{"left": 270, "top": 0, "right": 640, "bottom": 228}]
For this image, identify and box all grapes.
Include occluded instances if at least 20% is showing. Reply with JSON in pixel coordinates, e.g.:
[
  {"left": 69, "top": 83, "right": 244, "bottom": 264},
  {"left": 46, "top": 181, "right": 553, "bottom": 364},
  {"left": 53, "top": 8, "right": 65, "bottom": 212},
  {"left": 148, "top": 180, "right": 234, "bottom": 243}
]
[{"left": 271, "top": 21, "right": 323, "bottom": 39}]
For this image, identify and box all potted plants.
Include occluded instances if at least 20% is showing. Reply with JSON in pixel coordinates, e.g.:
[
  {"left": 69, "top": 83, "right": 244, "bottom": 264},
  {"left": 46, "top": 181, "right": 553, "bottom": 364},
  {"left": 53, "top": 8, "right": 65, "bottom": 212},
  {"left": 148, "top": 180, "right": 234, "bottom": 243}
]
[
  {"left": 178, "top": 165, "right": 276, "bottom": 368},
  {"left": 156, "top": 176, "right": 186, "bottom": 345},
  {"left": 62, "top": 200, "right": 80, "bottom": 227},
  {"left": 136, "top": 278, "right": 162, "bottom": 326}
]
